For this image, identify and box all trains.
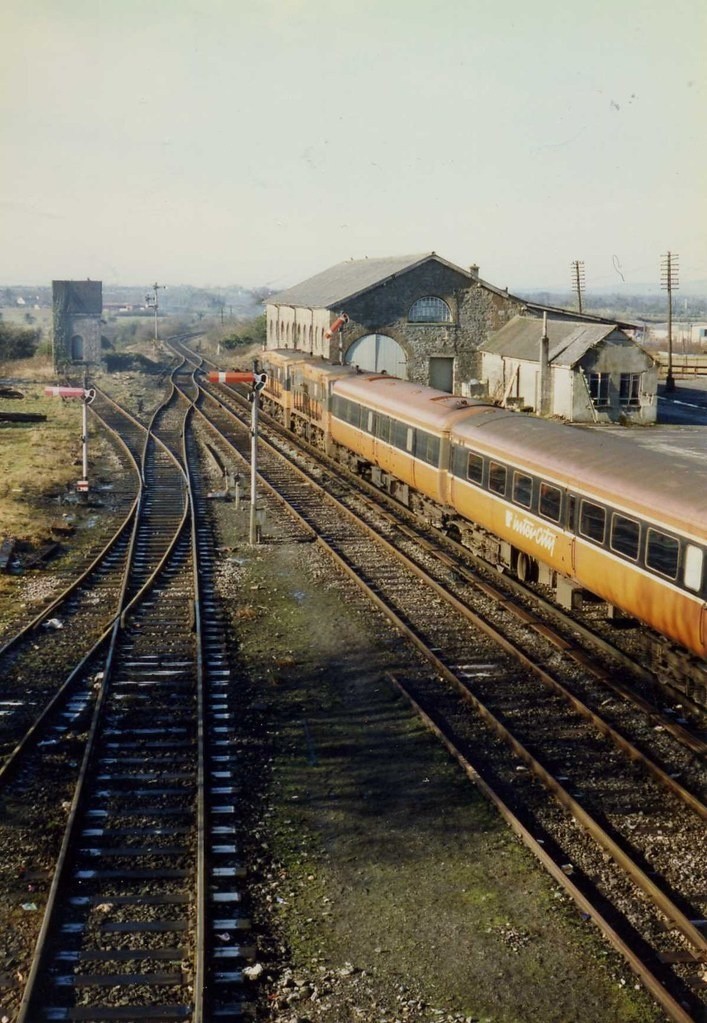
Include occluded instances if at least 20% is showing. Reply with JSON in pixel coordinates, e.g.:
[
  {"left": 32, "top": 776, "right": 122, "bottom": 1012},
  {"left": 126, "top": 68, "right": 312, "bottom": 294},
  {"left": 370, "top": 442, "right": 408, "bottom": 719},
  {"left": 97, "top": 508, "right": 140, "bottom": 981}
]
[{"left": 258, "top": 348, "right": 707, "bottom": 663}]
